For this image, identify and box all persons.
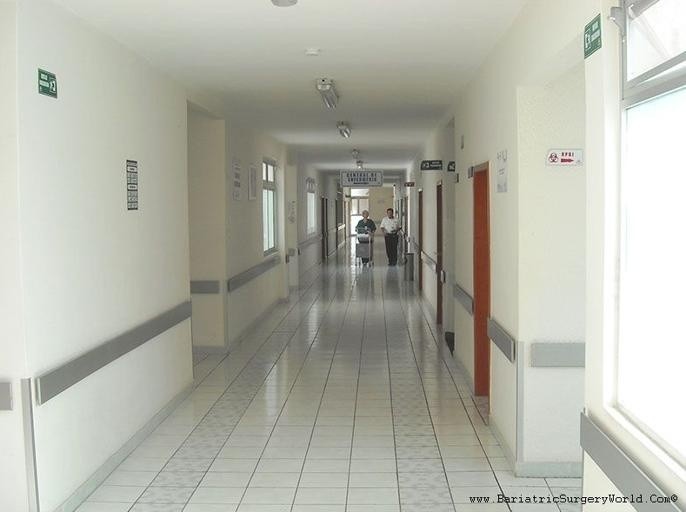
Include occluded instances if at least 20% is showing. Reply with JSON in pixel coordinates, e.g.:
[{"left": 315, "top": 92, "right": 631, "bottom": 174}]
[
  {"left": 355, "top": 210, "right": 376, "bottom": 266},
  {"left": 380, "top": 207, "right": 401, "bottom": 266}
]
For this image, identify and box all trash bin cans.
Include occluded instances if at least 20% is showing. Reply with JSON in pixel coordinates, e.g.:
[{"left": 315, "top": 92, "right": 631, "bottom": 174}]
[{"left": 404, "top": 253, "right": 414, "bottom": 282}]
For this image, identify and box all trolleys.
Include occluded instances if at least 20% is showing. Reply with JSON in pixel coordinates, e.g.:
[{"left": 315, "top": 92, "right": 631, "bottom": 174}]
[{"left": 355, "top": 228, "right": 374, "bottom": 267}]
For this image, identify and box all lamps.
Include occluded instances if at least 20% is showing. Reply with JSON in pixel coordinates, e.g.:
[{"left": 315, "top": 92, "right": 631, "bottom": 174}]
[
  {"left": 337, "top": 120, "right": 351, "bottom": 138},
  {"left": 356, "top": 160, "right": 363, "bottom": 166},
  {"left": 352, "top": 149, "right": 358, "bottom": 158},
  {"left": 316, "top": 78, "right": 337, "bottom": 110}
]
